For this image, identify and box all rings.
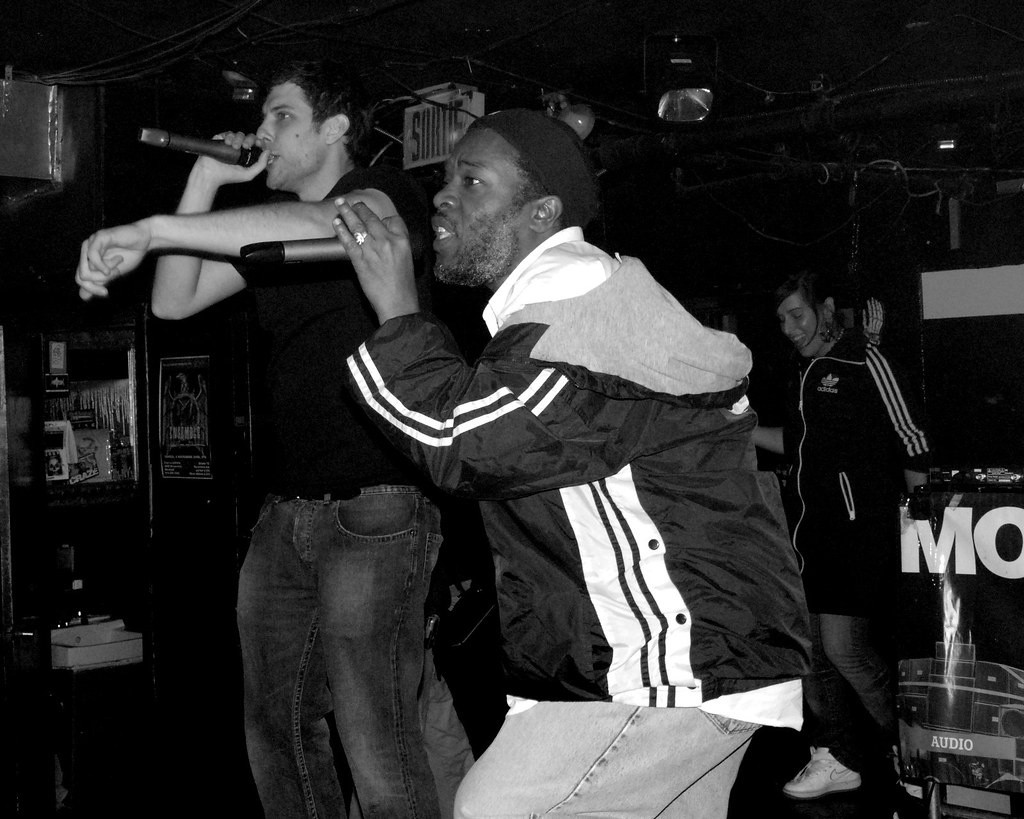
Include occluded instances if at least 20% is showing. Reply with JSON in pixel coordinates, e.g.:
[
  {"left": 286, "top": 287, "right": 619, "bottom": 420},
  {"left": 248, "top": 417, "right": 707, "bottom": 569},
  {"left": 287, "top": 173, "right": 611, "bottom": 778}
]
[{"left": 354, "top": 231, "right": 367, "bottom": 246}]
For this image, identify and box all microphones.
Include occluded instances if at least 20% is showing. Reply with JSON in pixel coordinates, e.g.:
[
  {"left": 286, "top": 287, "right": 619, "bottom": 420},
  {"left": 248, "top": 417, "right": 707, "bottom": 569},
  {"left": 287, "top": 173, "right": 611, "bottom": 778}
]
[
  {"left": 240, "top": 231, "right": 424, "bottom": 267},
  {"left": 138, "top": 127, "right": 262, "bottom": 168}
]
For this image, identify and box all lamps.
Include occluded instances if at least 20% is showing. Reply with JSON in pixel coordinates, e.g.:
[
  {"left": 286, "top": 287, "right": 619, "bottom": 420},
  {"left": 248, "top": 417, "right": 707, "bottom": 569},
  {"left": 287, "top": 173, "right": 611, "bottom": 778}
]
[
  {"left": 540, "top": 91, "right": 596, "bottom": 140},
  {"left": 650, "top": 68, "right": 720, "bottom": 130}
]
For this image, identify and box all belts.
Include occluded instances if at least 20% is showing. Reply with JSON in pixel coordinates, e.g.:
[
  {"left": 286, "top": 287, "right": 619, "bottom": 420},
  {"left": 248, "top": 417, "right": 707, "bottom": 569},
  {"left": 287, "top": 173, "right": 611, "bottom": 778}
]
[{"left": 266, "top": 481, "right": 362, "bottom": 502}]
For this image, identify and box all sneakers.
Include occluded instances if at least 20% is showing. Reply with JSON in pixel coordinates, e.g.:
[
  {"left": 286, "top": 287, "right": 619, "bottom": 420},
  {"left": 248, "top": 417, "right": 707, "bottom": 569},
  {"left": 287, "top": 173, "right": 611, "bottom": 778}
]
[{"left": 783, "top": 746, "right": 862, "bottom": 800}]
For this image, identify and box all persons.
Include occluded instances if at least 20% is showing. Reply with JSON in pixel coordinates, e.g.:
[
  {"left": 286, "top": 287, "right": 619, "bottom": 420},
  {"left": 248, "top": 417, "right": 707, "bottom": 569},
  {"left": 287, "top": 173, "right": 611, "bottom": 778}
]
[
  {"left": 333, "top": 109, "right": 816, "bottom": 819},
  {"left": 76, "top": 62, "right": 443, "bottom": 819},
  {"left": 772, "top": 267, "right": 931, "bottom": 798}
]
